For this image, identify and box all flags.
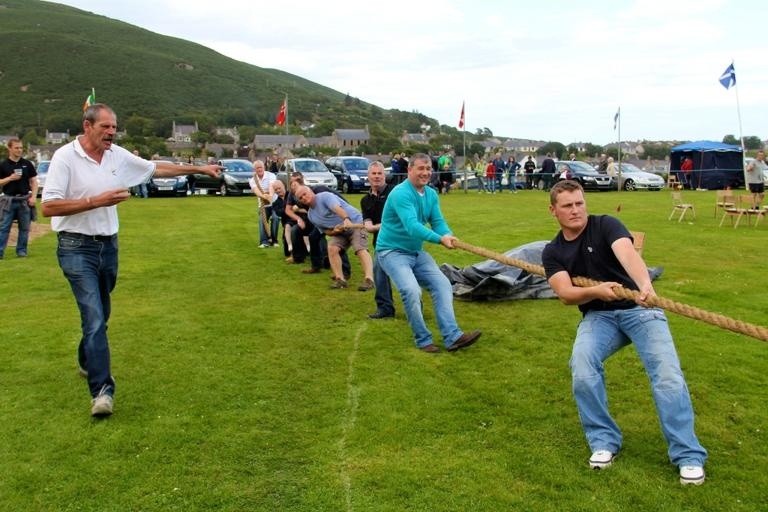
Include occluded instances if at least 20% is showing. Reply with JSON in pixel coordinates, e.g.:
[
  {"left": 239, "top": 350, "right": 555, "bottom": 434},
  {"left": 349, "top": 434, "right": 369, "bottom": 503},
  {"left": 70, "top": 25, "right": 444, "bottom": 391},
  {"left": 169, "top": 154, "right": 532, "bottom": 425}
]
[
  {"left": 458, "top": 103, "right": 464, "bottom": 128},
  {"left": 718, "top": 64, "right": 736, "bottom": 90},
  {"left": 276, "top": 98, "right": 286, "bottom": 126},
  {"left": 613, "top": 108, "right": 620, "bottom": 129},
  {"left": 82, "top": 91, "right": 95, "bottom": 112}
]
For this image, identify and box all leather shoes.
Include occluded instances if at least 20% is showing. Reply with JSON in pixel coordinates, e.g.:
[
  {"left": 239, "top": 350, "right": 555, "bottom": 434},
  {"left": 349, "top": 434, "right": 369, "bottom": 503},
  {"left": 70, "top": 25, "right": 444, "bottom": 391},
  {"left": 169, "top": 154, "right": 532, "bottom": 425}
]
[
  {"left": 421, "top": 344, "right": 440, "bottom": 353},
  {"left": 447, "top": 331, "right": 481, "bottom": 352}
]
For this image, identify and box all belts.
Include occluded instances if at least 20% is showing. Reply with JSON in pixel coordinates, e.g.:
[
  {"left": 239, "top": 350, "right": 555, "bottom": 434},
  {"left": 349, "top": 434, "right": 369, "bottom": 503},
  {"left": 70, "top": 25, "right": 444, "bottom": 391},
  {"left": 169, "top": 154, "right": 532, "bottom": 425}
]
[{"left": 61, "top": 231, "right": 118, "bottom": 242}]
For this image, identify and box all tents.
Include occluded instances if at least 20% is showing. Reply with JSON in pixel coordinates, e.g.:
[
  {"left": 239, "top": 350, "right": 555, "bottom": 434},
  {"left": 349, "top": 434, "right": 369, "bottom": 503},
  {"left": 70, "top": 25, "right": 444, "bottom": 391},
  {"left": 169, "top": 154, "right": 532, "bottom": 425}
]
[{"left": 671, "top": 140, "right": 746, "bottom": 191}]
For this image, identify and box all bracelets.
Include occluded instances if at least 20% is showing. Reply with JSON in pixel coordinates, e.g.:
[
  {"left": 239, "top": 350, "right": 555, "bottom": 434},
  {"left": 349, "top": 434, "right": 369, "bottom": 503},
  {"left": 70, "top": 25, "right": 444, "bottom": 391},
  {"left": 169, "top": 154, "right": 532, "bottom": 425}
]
[{"left": 87, "top": 198, "right": 91, "bottom": 207}]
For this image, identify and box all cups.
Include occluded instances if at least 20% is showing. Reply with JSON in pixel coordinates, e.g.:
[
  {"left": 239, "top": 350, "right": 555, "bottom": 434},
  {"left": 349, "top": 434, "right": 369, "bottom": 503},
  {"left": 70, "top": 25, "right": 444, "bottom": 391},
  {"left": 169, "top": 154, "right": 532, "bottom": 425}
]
[
  {"left": 118, "top": 184, "right": 129, "bottom": 201},
  {"left": 14, "top": 168, "right": 23, "bottom": 177}
]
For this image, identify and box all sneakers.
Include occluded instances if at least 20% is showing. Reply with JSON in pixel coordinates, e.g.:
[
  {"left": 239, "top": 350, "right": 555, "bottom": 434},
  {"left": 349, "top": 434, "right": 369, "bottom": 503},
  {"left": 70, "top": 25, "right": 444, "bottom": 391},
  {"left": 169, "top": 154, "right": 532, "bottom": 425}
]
[
  {"left": 358, "top": 280, "right": 374, "bottom": 291},
  {"left": 257, "top": 243, "right": 271, "bottom": 249},
  {"left": 589, "top": 448, "right": 617, "bottom": 469},
  {"left": 273, "top": 243, "right": 280, "bottom": 248},
  {"left": 369, "top": 309, "right": 395, "bottom": 318},
  {"left": 329, "top": 280, "right": 346, "bottom": 289},
  {"left": 302, "top": 267, "right": 322, "bottom": 273},
  {"left": 17, "top": 251, "right": 27, "bottom": 257},
  {"left": 80, "top": 368, "right": 114, "bottom": 382},
  {"left": 330, "top": 274, "right": 351, "bottom": 281},
  {"left": 91, "top": 384, "right": 113, "bottom": 417},
  {"left": 679, "top": 463, "right": 705, "bottom": 487},
  {"left": 288, "top": 258, "right": 304, "bottom": 264}
]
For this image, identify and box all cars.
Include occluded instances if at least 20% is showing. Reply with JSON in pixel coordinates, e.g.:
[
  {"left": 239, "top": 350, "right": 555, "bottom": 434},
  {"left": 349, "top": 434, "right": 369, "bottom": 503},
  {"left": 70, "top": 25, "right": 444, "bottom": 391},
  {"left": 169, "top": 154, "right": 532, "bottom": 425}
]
[
  {"left": 325, "top": 157, "right": 370, "bottom": 194},
  {"left": 530, "top": 160, "right": 616, "bottom": 192},
  {"left": 127, "top": 159, "right": 257, "bottom": 197},
  {"left": 593, "top": 163, "right": 665, "bottom": 191},
  {"left": 277, "top": 158, "right": 337, "bottom": 192},
  {"left": 30, "top": 160, "right": 51, "bottom": 197}
]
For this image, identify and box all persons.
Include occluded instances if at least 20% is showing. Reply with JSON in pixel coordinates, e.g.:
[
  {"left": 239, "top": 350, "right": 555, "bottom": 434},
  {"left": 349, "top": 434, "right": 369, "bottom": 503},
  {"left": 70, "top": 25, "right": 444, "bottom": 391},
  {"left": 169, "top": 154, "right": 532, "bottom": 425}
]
[
  {"left": 680, "top": 156, "right": 694, "bottom": 190},
  {"left": 599, "top": 154, "right": 618, "bottom": 179},
  {"left": 392, "top": 149, "right": 454, "bottom": 193},
  {"left": 361, "top": 161, "right": 396, "bottom": 319},
  {"left": 248, "top": 155, "right": 375, "bottom": 291},
  {"left": 40, "top": 102, "right": 228, "bottom": 417},
  {"left": 541, "top": 179, "right": 709, "bottom": 486},
  {"left": 375, "top": 153, "right": 482, "bottom": 353},
  {"left": 186, "top": 154, "right": 198, "bottom": 195},
  {"left": 747, "top": 150, "right": 768, "bottom": 210},
  {"left": 477, "top": 153, "right": 572, "bottom": 194},
  {"left": 0, "top": 139, "right": 38, "bottom": 259},
  {"left": 130, "top": 150, "right": 150, "bottom": 198}
]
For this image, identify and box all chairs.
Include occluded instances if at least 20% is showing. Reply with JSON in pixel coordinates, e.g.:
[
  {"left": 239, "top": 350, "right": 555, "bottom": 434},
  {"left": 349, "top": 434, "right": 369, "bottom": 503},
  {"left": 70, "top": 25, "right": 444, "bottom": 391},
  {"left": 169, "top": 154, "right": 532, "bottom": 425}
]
[
  {"left": 739, "top": 195, "right": 768, "bottom": 228},
  {"left": 669, "top": 181, "right": 696, "bottom": 223},
  {"left": 714, "top": 189, "right": 734, "bottom": 218},
  {"left": 667, "top": 175, "right": 679, "bottom": 190},
  {"left": 719, "top": 187, "right": 745, "bottom": 228}
]
